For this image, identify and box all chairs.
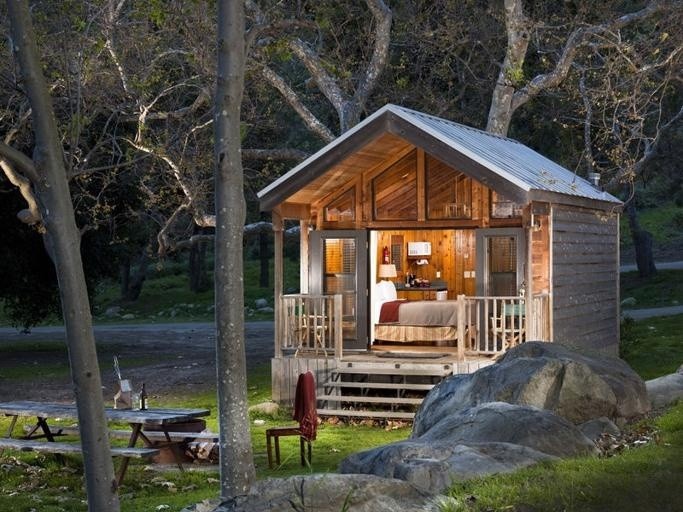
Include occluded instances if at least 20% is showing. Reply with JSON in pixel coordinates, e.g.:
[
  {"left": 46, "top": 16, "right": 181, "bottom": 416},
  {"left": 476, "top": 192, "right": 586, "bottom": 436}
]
[
  {"left": 0, "top": 424, "right": 218, "bottom": 488},
  {"left": 264, "top": 371, "right": 318, "bottom": 470}
]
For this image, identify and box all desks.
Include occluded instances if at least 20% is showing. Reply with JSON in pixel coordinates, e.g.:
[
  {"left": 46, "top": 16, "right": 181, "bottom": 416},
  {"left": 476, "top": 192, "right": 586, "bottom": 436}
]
[{"left": 0, "top": 400, "right": 211, "bottom": 485}]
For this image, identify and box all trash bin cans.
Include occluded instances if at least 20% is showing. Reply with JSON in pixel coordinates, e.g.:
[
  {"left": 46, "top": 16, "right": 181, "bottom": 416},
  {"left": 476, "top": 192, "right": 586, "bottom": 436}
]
[{"left": 506, "top": 316, "right": 525, "bottom": 342}]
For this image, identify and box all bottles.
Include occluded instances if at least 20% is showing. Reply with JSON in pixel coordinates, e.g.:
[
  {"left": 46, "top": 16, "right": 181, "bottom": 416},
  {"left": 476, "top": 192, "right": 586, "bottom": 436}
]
[
  {"left": 139, "top": 383, "right": 148, "bottom": 411},
  {"left": 383, "top": 246, "right": 390, "bottom": 264}
]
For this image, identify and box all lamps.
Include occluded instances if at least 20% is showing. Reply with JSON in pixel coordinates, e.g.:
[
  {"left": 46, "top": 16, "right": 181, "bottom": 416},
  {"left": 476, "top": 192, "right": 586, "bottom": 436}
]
[{"left": 379, "top": 265, "right": 396, "bottom": 282}]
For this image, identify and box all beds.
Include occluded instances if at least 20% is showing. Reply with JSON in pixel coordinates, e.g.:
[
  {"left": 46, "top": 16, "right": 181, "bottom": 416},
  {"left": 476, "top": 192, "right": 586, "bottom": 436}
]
[{"left": 374, "top": 280, "right": 477, "bottom": 347}]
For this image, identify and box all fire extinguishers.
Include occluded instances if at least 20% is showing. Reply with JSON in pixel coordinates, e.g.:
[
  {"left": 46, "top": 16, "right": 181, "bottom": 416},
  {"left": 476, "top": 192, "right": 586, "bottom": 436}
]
[{"left": 384, "top": 246, "right": 390, "bottom": 265}]
